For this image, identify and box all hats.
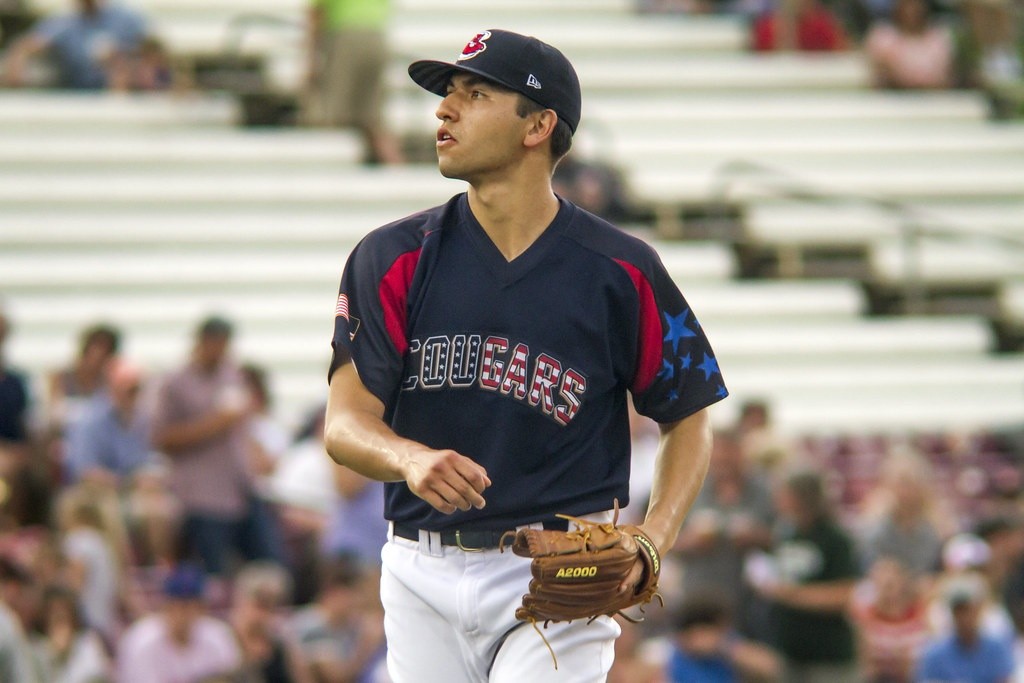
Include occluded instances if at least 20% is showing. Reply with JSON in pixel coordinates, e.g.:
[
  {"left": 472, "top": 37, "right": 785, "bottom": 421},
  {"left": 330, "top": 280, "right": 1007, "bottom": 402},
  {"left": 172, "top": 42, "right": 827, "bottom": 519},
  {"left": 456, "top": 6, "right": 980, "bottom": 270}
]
[{"left": 407, "top": 30, "right": 582, "bottom": 136}]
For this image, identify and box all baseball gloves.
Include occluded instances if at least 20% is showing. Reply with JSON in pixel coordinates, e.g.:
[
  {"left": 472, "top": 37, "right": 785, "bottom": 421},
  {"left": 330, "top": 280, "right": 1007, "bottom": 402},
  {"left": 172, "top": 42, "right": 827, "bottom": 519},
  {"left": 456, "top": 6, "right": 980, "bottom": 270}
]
[{"left": 498, "top": 521, "right": 662, "bottom": 623}]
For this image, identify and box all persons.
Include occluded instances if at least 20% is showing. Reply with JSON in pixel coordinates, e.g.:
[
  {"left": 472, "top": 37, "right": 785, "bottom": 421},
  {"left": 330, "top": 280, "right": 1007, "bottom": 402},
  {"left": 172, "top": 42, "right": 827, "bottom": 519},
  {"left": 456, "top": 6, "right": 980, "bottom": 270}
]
[
  {"left": 604, "top": 396, "right": 1024, "bottom": 683},
  {"left": 750, "top": 0, "right": 958, "bottom": 88},
  {"left": 0, "top": 0, "right": 408, "bottom": 163},
  {"left": 323, "top": 29, "right": 729, "bottom": 683},
  {"left": 0, "top": 310, "right": 390, "bottom": 683}
]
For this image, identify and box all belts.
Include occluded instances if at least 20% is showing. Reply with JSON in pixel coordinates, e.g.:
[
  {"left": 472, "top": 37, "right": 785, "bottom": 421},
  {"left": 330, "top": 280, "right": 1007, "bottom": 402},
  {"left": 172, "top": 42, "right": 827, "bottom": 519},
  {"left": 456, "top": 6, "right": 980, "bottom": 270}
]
[{"left": 393, "top": 521, "right": 571, "bottom": 551}]
